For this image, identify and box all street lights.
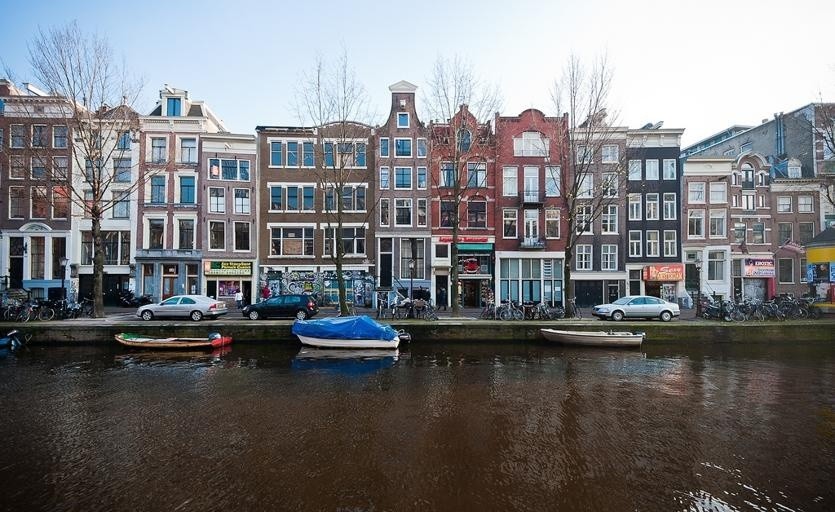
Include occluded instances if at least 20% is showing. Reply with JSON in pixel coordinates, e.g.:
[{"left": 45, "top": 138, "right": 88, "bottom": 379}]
[
  {"left": 407, "top": 259, "right": 417, "bottom": 318},
  {"left": 59, "top": 256, "right": 69, "bottom": 300},
  {"left": 694, "top": 258, "right": 704, "bottom": 318}
]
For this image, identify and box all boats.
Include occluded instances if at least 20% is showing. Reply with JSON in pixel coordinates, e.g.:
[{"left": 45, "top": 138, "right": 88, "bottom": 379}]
[
  {"left": 540, "top": 328, "right": 646, "bottom": 348},
  {"left": 296, "top": 334, "right": 400, "bottom": 349},
  {"left": 114, "top": 332, "right": 233, "bottom": 348}
]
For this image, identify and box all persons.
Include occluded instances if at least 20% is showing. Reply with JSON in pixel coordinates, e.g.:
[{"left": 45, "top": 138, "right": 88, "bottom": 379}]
[
  {"left": 813, "top": 279, "right": 831, "bottom": 302},
  {"left": 261, "top": 286, "right": 270, "bottom": 301},
  {"left": 438, "top": 286, "right": 447, "bottom": 311},
  {"left": 235, "top": 288, "right": 244, "bottom": 309}
]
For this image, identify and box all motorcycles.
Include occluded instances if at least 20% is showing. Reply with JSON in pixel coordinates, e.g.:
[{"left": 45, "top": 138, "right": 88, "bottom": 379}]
[{"left": 87, "top": 287, "right": 153, "bottom": 308}]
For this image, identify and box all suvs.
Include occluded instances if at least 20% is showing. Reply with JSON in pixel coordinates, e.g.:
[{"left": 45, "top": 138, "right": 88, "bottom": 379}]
[{"left": 241, "top": 293, "right": 320, "bottom": 320}]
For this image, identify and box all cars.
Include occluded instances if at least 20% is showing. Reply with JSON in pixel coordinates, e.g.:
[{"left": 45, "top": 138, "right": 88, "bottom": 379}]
[
  {"left": 136, "top": 294, "right": 228, "bottom": 322},
  {"left": 591, "top": 295, "right": 680, "bottom": 322}
]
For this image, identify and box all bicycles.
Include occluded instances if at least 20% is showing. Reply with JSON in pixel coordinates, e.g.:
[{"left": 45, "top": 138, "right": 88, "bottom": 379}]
[
  {"left": 479, "top": 297, "right": 582, "bottom": 321},
  {"left": 699, "top": 293, "right": 821, "bottom": 322},
  {"left": 0, "top": 297, "right": 93, "bottom": 323},
  {"left": 376, "top": 295, "right": 440, "bottom": 321}
]
